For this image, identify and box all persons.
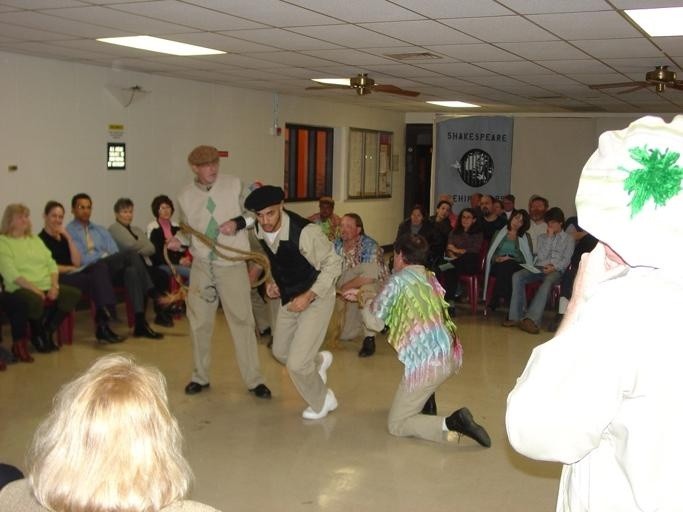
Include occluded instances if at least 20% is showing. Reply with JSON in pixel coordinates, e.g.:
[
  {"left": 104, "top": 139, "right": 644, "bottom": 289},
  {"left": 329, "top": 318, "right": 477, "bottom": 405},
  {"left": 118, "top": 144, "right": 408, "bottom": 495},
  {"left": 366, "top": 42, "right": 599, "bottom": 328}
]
[
  {"left": 342, "top": 234, "right": 491, "bottom": 447},
  {"left": 244, "top": 185, "right": 342, "bottom": 420},
  {"left": 0, "top": 354, "right": 221, "bottom": 512},
  {"left": 0, "top": 193, "right": 193, "bottom": 371},
  {"left": 246, "top": 227, "right": 272, "bottom": 344},
  {"left": 307, "top": 197, "right": 341, "bottom": 241},
  {"left": 504, "top": 116, "right": 683, "bottom": 512},
  {"left": 334, "top": 214, "right": 390, "bottom": 357},
  {"left": 176, "top": 146, "right": 271, "bottom": 398},
  {"left": 389, "top": 193, "right": 599, "bottom": 334}
]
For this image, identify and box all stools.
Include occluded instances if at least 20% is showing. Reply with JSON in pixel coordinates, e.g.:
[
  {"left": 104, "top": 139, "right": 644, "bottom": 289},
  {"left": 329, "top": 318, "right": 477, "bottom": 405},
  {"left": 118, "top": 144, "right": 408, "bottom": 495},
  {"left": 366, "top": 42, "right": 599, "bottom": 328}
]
[{"left": 0, "top": 272, "right": 186, "bottom": 351}]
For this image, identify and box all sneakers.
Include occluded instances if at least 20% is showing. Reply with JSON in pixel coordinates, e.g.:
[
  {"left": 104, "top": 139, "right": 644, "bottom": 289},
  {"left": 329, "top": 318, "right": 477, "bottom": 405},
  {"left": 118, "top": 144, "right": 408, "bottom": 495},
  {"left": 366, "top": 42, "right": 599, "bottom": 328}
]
[
  {"left": 316, "top": 350, "right": 333, "bottom": 383},
  {"left": 301, "top": 389, "right": 339, "bottom": 420}
]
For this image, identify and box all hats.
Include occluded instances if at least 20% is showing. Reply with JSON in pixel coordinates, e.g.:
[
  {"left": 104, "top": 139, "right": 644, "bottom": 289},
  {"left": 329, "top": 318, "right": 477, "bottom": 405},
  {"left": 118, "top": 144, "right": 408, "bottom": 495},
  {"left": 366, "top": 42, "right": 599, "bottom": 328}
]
[
  {"left": 188, "top": 145, "right": 221, "bottom": 167},
  {"left": 319, "top": 197, "right": 333, "bottom": 204},
  {"left": 244, "top": 184, "right": 285, "bottom": 212}
]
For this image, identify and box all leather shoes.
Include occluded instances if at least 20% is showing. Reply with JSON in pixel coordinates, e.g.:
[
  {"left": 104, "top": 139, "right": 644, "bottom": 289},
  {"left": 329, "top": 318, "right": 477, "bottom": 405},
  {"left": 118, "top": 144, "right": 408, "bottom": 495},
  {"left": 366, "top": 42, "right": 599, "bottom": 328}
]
[
  {"left": 502, "top": 317, "right": 516, "bottom": 327},
  {"left": 421, "top": 391, "right": 438, "bottom": 415},
  {"left": 445, "top": 408, "right": 491, "bottom": 449},
  {"left": 248, "top": 383, "right": 272, "bottom": 399},
  {"left": 359, "top": 335, "right": 375, "bottom": 359},
  {"left": 547, "top": 317, "right": 563, "bottom": 332},
  {"left": 185, "top": 380, "right": 210, "bottom": 395},
  {"left": 260, "top": 326, "right": 271, "bottom": 336},
  {"left": 518, "top": 317, "right": 539, "bottom": 335},
  {"left": 1, "top": 293, "right": 185, "bottom": 370},
  {"left": 382, "top": 325, "right": 390, "bottom": 335},
  {"left": 453, "top": 289, "right": 464, "bottom": 299}
]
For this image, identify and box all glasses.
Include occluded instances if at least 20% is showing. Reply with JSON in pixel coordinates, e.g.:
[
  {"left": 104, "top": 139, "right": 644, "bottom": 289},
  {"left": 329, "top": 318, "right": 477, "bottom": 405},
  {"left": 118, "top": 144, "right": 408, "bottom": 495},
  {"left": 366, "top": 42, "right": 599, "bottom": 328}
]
[{"left": 76, "top": 205, "right": 91, "bottom": 209}]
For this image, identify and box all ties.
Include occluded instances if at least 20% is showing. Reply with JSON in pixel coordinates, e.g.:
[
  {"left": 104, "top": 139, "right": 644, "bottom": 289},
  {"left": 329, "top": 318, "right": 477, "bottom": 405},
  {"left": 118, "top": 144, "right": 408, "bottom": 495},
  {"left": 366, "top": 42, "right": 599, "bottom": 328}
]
[{"left": 82, "top": 224, "right": 94, "bottom": 256}]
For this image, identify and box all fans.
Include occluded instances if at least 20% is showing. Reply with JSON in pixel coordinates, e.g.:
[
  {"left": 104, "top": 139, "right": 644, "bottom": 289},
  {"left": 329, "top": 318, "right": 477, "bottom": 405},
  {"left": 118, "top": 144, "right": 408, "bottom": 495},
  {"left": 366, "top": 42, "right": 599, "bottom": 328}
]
[
  {"left": 301, "top": 73, "right": 424, "bottom": 98},
  {"left": 582, "top": 63, "right": 682, "bottom": 97}
]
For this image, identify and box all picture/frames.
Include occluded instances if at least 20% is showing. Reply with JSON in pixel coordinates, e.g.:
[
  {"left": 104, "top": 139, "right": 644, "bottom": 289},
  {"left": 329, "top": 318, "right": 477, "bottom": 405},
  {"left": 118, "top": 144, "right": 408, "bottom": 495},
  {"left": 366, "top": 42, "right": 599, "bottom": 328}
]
[{"left": 343, "top": 122, "right": 394, "bottom": 200}]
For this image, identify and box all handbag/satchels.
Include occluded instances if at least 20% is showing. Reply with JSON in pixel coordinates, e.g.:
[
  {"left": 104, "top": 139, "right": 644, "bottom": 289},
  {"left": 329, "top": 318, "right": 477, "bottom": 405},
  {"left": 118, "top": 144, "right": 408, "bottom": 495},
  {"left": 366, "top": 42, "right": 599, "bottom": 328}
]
[{"left": 490, "top": 254, "right": 512, "bottom": 279}]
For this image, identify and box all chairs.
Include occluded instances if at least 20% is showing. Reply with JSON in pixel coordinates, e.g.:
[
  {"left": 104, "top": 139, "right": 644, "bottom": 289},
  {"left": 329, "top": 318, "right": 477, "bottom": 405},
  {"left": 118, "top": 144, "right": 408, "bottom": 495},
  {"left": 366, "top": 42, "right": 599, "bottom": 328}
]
[{"left": 437, "top": 236, "right": 573, "bottom": 317}]
